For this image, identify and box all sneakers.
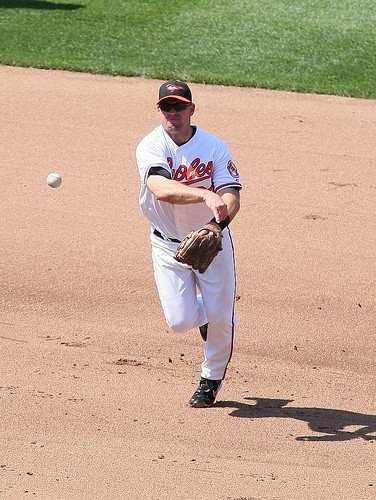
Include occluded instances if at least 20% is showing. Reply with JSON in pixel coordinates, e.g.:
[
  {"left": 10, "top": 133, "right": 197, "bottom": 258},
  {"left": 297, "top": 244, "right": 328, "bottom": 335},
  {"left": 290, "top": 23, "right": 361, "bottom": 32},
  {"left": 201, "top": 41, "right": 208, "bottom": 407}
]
[
  {"left": 199, "top": 322, "right": 209, "bottom": 342},
  {"left": 188, "top": 376, "right": 224, "bottom": 408}
]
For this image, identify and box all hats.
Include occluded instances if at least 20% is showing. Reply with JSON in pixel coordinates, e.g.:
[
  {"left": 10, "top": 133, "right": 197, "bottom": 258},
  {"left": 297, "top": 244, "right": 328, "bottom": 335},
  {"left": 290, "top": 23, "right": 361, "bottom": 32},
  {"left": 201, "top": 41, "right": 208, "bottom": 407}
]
[{"left": 156, "top": 79, "right": 192, "bottom": 104}]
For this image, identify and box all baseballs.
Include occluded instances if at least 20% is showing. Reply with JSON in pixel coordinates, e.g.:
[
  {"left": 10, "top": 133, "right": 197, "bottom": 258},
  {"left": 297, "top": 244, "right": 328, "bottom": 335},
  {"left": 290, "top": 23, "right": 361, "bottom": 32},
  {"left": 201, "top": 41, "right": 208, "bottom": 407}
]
[{"left": 46, "top": 172, "right": 63, "bottom": 188}]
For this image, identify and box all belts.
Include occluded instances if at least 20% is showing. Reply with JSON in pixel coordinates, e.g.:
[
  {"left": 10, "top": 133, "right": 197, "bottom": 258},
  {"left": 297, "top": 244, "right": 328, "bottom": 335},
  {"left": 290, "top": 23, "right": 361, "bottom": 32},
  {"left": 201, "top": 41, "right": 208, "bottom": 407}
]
[{"left": 152, "top": 230, "right": 182, "bottom": 243}]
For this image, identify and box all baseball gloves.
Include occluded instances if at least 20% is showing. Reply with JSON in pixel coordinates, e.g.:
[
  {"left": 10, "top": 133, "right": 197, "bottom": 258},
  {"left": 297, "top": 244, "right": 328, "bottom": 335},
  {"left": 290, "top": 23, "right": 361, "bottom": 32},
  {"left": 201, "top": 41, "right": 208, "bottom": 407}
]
[{"left": 172, "top": 221, "right": 224, "bottom": 274}]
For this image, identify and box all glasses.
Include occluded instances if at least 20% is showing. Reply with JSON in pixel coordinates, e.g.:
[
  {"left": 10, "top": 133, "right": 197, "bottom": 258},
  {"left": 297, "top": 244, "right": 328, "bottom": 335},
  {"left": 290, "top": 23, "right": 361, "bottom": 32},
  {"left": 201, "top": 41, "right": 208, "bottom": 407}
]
[{"left": 159, "top": 101, "right": 190, "bottom": 112}]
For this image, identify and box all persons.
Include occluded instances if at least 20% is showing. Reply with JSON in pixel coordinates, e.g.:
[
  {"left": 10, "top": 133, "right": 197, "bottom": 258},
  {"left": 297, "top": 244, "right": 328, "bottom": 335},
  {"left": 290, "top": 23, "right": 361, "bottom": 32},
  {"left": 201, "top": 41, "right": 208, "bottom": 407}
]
[{"left": 137, "top": 81, "right": 241, "bottom": 409}]
